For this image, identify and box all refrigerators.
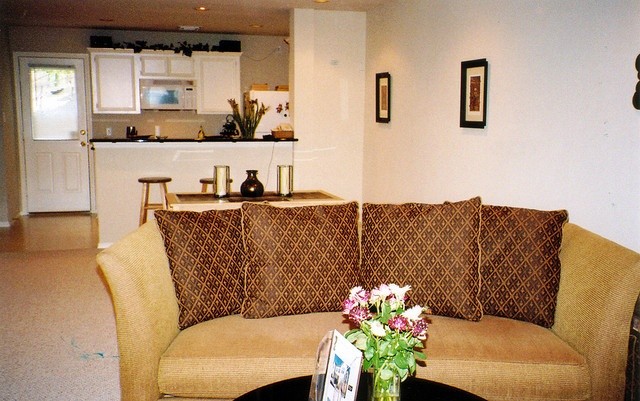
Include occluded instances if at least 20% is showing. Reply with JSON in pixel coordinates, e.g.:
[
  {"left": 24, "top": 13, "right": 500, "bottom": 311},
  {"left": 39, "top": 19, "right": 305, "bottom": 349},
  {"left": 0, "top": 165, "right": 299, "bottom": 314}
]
[{"left": 243, "top": 90, "right": 290, "bottom": 138}]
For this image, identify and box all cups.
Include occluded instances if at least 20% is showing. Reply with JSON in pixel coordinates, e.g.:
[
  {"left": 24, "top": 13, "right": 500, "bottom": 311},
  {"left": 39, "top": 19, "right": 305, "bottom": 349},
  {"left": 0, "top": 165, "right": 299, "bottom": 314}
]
[
  {"left": 277, "top": 165, "right": 294, "bottom": 196},
  {"left": 213, "top": 164, "right": 231, "bottom": 198}
]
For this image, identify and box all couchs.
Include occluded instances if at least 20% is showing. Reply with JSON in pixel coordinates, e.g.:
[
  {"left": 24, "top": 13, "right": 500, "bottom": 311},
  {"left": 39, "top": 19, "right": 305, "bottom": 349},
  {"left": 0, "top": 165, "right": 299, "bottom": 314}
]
[{"left": 96, "top": 203, "right": 640, "bottom": 401}]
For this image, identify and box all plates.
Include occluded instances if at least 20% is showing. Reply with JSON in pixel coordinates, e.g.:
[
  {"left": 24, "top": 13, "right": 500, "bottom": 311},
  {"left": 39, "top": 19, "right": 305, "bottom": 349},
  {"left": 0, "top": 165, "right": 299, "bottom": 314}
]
[{"left": 156, "top": 135, "right": 168, "bottom": 140}]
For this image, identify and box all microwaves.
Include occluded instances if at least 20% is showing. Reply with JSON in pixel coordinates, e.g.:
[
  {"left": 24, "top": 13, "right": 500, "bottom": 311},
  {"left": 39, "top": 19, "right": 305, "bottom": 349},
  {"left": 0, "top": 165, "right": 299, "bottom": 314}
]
[{"left": 141, "top": 79, "right": 197, "bottom": 111}]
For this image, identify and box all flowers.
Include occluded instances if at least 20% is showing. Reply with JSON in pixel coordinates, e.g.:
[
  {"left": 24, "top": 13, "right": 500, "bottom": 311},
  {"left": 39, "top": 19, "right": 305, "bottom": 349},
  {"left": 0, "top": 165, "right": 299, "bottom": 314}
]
[
  {"left": 341, "top": 284, "right": 431, "bottom": 401},
  {"left": 228, "top": 92, "right": 269, "bottom": 139}
]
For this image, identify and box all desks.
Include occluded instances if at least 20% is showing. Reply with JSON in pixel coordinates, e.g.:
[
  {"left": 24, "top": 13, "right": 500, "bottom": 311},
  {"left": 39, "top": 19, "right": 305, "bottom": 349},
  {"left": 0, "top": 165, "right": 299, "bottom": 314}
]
[
  {"left": 233, "top": 372, "right": 486, "bottom": 401},
  {"left": 164, "top": 190, "right": 345, "bottom": 211}
]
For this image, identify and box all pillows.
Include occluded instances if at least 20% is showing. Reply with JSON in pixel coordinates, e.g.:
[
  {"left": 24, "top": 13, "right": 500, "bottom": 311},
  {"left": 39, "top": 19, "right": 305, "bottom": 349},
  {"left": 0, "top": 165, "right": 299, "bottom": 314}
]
[
  {"left": 445, "top": 200, "right": 569, "bottom": 328},
  {"left": 153, "top": 208, "right": 244, "bottom": 330},
  {"left": 360, "top": 197, "right": 483, "bottom": 322},
  {"left": 241, "top": 201, "right": 361, "bottom": 319}
]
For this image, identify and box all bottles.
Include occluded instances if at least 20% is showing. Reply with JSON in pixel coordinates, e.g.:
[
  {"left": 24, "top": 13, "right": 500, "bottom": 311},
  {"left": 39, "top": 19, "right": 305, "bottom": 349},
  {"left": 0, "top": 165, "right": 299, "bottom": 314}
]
[
  {"left": 155, "top": 124, "right": 161, "bottom": 139},
  {"left": 198, "top": 125, "right": 205, "bottom": 139}
]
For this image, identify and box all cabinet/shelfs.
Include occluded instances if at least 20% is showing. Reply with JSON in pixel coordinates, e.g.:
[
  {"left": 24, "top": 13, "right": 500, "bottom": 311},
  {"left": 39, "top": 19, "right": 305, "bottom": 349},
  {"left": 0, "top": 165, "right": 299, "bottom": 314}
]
[
  {"left": 91, "top": 53, "right": 141, "bottom": 115},
  {"left": 139, "top": 55, "right": 197, "bottom": 110},
  {"left": 195, "top": 56, "right": 242, "bottom": 115}
]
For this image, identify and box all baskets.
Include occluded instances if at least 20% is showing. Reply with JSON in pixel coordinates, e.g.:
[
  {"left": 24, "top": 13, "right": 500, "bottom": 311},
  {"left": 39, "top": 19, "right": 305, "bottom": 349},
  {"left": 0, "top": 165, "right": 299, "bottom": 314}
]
[{"left": 270, "top": 129, "right": 294, "bottom": 138}]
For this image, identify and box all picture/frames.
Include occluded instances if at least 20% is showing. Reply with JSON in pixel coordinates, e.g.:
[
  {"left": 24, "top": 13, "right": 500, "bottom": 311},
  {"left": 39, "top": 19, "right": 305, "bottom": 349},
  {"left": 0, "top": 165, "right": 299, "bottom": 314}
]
[
  {"left": 460, "top": 59, "right": 487, "bottom": 128},
  {"left": 376, "top": 72, "right": 391, "bottom": 123}
]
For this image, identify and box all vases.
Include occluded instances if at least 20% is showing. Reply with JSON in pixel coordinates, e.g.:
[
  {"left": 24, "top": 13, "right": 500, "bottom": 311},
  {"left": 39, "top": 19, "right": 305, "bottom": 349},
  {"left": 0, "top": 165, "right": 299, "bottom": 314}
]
[
  {"left": 369, "top": 366, "right": 400, "bottom": 401},
  {"left": 241, "top": 170, "right": 264, "bottom": 197}
]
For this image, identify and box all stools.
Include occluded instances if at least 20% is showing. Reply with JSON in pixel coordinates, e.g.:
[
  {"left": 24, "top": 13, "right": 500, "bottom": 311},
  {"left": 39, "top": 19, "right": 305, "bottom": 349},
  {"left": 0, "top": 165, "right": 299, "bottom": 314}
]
[
  {"left": 200, "top": 178, "right": 233, "bottom": 194},
  {"left": 139, "top": 177, "right": 172, "bottom": 227}
]
